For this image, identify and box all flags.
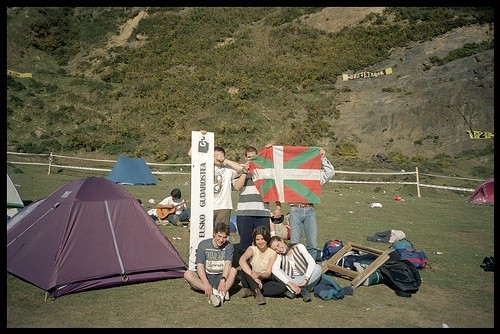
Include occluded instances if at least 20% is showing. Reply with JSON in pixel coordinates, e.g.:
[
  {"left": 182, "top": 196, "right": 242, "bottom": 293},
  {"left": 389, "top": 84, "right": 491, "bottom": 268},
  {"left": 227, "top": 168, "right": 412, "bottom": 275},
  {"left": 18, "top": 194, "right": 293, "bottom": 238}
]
[{"left": 248, "top": 145, "right": 322, "bottom": 205}]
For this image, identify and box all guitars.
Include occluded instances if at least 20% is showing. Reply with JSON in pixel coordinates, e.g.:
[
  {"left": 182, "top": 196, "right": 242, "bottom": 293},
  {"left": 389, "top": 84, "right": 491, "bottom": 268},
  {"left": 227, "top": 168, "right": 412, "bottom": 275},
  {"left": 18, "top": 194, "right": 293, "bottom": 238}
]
[{"left": 156, "top": 200, "right": 190, "bottom": 219}]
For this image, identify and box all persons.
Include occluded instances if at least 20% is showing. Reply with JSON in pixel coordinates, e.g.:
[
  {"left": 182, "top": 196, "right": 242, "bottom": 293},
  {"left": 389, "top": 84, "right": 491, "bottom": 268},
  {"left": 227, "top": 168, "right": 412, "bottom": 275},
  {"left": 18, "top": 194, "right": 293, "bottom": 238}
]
[
  {"left": 188, "top": 130, "right": 244, "bottom": 226},
  {"left": 232, "top": 147, "right": 283, "bottom": 266},
  {"left": 268, "top": 236, "right": 323, "bottom": 302},
  {"left": 156, "top": 188, "right": 191, "bottom": 226},
  {"left": 184, "top": 222, "right": 237, "bottom": 307},
  {"left": 239, "top": 226, "right": 286, "bottom": 305},
  {"left": 289, "top": 148, "right": 335, "bottom": 263},
  {"left": 270, "top": 212, "right": 291, "bottom": 240}
]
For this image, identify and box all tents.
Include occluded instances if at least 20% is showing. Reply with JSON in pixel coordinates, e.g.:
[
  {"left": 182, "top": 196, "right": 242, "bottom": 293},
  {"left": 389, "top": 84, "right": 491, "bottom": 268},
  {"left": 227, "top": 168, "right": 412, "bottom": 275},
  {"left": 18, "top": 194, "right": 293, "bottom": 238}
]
[
  {"left": 7, "top": 176, "right": 187, "bottom": 300},
  {"left": 467, "top": 178, "right": 494, "bottom": 206},
  {"left": 105, "top": 155, "right": 157, "bottom": 187}
]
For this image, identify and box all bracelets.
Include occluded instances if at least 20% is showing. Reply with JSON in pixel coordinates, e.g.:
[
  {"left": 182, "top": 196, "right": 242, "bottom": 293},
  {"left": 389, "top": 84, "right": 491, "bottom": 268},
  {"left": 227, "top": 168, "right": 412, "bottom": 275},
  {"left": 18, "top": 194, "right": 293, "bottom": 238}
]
[
  {"left": 222, "top": 158, "right": 226, "bottom": 163},
  {"left": 277, "top": 207, "right": 282, "bottom": 210}
]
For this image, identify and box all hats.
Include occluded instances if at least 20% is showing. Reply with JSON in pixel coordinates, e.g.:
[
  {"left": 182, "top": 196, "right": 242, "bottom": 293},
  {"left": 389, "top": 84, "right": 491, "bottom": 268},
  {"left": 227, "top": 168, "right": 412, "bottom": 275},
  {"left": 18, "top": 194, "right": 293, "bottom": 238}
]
[{"left": 270, "top": 215, "right": 284, "bottom": 224}]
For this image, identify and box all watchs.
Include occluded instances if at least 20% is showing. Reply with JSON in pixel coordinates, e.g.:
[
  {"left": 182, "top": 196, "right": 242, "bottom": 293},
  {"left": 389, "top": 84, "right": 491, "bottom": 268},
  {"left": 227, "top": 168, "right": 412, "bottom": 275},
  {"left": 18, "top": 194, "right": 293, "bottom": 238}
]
[
  {"left": 221, "top": 276, "right": 226, "bottom": 282},
  {"left": 304, "top": 277, "right": 308, "bottom": 282}
]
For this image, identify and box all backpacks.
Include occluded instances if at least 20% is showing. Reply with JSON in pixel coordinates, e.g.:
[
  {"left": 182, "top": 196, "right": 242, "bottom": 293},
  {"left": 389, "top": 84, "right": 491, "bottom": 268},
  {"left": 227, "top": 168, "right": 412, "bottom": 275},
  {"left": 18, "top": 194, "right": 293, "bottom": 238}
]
[{"left": 322, "top": 240, "right": 343, "bottom": 261}]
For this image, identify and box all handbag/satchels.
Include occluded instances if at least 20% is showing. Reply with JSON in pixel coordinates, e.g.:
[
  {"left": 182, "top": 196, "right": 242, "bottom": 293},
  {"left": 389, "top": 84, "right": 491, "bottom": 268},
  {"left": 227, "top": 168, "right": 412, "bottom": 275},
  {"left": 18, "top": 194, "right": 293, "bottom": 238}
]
[
  {"left": 353, "top": 262, "right": 382, "bottom": 286},
  {"left": 392, "top": 240, "right": 412, "bottom": 252},
  {"left": 396, "top": 249, "right": 427, "bottom": 269}
]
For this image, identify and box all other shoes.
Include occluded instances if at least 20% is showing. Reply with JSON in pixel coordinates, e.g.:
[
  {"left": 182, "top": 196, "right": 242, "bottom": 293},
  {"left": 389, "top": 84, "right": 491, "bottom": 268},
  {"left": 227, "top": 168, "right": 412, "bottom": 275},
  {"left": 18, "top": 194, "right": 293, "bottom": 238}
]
[
  {"left": 286, "top": 290, "right": 295, "bottom": 299},
  {"left": 256, "top": 294, "right": 266, "bottom": 304},
  {"left": 303, "top": 295, "right": 312, "bottom": 302},
  {"left": 210, "top": 294, "right": 220, "bottom": 307},
  {"left": 242, "top": 287, "right": 251, "bottom": 298},
  {"left": 225, "top": 292, "right": 230, "bottom": 301}
]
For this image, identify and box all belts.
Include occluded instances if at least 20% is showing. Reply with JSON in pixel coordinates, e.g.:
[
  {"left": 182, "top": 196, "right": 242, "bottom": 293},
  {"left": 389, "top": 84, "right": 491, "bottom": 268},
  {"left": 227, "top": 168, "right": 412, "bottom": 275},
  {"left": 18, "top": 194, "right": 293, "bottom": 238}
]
[{"left": 290, "top": 205, "right": 312, "bottom": 207}]
